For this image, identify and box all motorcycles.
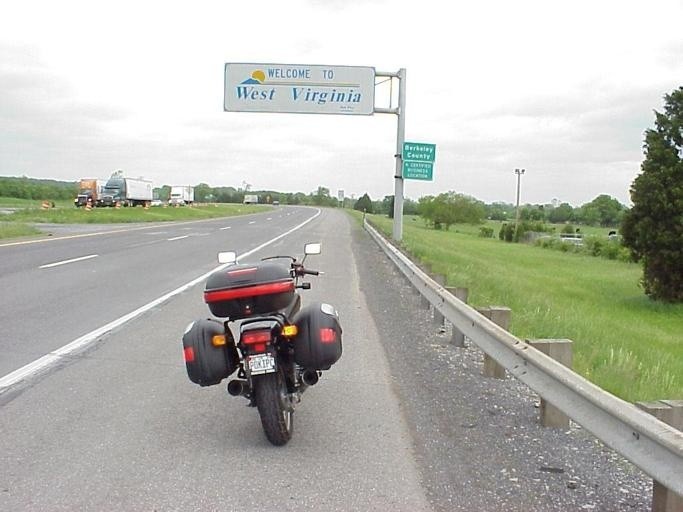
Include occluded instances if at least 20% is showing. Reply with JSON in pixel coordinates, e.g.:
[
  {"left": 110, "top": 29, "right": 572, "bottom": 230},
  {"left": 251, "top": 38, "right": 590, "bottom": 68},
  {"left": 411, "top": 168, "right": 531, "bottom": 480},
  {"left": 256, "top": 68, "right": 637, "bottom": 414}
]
[{"left": 182, "top": 242, "right": 342, "bottom": 446}]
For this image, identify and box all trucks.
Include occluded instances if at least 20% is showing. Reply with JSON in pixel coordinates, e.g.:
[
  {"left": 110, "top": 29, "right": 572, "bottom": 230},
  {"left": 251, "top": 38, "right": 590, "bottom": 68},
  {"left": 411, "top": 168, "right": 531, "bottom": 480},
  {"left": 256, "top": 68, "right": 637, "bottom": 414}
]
[
  {"left": 242, "top": 194, "right": 258, "bottom": 205},
  {"left": 74, "top": 176, "right": 152, "bottom": 208},
  {"left": 168, "top": 186, "right": 195, "bottom": 206}
]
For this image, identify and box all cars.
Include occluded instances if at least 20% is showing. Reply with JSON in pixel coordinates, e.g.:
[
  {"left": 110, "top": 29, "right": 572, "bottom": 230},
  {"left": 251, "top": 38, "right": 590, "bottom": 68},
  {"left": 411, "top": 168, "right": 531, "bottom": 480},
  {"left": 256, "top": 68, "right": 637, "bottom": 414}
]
[
  {"left": 272, "top": 200, "right": 279, "bottom": 205},
  {"left": 169, "top": 199, "right": 186, "bottom": 206},
  {"left": 151, "top": 200, "right": 164, "bottom": 207}
]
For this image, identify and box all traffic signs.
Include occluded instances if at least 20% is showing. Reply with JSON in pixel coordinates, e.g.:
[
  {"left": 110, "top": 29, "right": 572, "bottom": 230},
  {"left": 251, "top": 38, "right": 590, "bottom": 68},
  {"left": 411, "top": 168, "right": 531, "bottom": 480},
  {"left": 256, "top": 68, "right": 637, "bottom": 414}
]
[{"left": 224, "top": 59, "right": 376, "bottom": 115}]
